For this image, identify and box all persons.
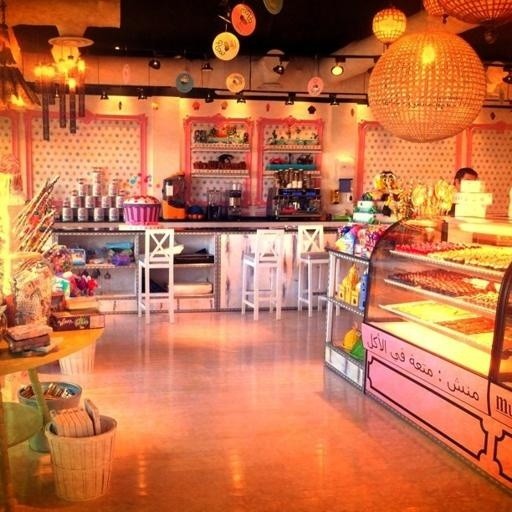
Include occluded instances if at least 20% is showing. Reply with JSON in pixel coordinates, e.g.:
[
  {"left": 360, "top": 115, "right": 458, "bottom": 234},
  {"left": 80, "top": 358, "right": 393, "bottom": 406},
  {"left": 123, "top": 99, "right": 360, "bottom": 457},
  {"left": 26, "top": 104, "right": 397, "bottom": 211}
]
[{"left": 449, "top": 167, "right": 478, "bottom": 217}]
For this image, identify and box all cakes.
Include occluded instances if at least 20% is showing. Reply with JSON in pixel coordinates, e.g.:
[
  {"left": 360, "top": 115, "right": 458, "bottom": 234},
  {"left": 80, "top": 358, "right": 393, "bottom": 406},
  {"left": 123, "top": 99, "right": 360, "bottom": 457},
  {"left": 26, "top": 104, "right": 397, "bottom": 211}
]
[{"left": 62, "top": 171, "right": 125, "bottom": 223}]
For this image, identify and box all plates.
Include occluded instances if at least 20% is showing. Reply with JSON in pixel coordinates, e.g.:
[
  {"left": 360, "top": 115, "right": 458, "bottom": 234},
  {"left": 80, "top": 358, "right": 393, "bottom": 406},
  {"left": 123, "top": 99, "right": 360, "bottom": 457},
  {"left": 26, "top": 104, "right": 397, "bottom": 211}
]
[
  {"left": 43, "top": 220, "right": 354, "bottom": 315},
  {"left": 185, "top": 115, "right": 324, "bottom": 208}
]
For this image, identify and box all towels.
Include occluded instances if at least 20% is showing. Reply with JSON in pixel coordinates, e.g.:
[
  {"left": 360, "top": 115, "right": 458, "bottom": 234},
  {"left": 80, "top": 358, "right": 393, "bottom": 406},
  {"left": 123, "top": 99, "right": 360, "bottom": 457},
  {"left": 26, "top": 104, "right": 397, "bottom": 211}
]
[{"left": 17, "top": 382, "right": 119, "bottom": 502}]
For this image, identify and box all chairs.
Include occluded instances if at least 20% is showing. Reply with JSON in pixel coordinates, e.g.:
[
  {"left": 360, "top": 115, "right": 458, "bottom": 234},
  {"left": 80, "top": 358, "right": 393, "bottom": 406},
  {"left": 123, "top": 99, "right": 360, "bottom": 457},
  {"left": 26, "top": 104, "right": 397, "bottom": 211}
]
[
  {"left": 137, "top": 229, "right": 173, "bottom": 323},
  {"left": 241, "top": 225, "right": 329, "bottom": 319}
]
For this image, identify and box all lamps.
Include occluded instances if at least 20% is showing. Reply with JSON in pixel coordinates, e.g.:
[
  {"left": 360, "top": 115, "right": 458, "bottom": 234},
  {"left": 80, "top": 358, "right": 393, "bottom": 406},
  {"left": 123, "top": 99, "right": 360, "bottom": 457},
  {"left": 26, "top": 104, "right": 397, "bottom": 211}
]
[
  {"left": 241, "top": 225, "right": 329, "bottom": 319},
  {"left": 137, "top": 229, "right": 173, "bottom": 323},
  {"left": 0, "top": 0, "right": 512, "bottom": 142}
]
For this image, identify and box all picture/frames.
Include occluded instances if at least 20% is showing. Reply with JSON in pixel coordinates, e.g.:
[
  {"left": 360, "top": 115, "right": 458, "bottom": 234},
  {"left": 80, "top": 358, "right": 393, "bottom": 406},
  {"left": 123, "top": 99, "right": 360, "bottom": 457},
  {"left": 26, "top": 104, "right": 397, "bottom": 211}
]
[{"left": 1, "top": 328, "right": 105, "bottom": 428}]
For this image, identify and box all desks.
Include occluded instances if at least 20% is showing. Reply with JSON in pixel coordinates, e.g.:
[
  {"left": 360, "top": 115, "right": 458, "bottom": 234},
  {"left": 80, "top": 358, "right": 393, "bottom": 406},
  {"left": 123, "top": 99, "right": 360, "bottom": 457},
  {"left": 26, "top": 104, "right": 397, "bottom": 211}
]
[{"left": 1, "top": 328, "right": 105, "bottom": 428}]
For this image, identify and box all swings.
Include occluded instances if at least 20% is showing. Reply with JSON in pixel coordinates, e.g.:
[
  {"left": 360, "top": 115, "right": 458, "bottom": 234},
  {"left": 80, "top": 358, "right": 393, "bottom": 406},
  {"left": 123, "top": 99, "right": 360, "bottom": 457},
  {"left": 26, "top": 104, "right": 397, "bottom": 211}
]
[{"left": 333, "top": 177, "right": 353, "bottom": 203}]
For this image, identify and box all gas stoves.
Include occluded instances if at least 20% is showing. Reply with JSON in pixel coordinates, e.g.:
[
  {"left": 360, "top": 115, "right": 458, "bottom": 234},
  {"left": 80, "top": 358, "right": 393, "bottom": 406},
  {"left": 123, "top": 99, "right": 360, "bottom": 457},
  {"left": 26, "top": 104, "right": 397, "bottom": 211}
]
[{"left": 389, "top": 241, "right": 512, "bottom": 311}]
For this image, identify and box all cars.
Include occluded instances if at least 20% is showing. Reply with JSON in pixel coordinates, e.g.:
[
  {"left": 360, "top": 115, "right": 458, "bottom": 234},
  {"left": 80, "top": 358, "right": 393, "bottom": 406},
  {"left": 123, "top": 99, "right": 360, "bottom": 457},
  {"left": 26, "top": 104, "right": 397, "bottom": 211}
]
[{"left": 208, "top": 191, "right": 222, "bottom": 221}]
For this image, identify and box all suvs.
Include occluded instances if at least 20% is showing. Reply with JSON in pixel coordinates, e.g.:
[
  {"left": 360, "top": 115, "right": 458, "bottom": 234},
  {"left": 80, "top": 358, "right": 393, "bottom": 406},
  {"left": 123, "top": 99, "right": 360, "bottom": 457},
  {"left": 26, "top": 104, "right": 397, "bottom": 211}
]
[
  {"left": 19, "top": 381, "right": 83, "bottom": 452},
  {"left": 44, "top": 412, "right": 116, "bottom": 501}
]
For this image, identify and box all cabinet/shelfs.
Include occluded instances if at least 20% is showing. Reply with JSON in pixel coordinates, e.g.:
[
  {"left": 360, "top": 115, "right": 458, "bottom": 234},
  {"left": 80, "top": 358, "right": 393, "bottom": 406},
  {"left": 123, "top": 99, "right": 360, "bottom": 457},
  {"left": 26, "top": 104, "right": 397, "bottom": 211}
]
[
  {"left": 274, "top": 168, "right": 316, "bottom": 191},
  {"left": 227, "top": 190, "right": 242, "bottom": 221},
  {"left": 366, "top": 218, "right": 512, "bottom": 494},
  {"left": 325, "top": 217, "right": 405, "bottom": 392},
  {"left": 43, "top": 220, "right": 354, "bottom": 315},
  {"left": 185, "top": 115, "right": 324, "bottom": 208}
]
